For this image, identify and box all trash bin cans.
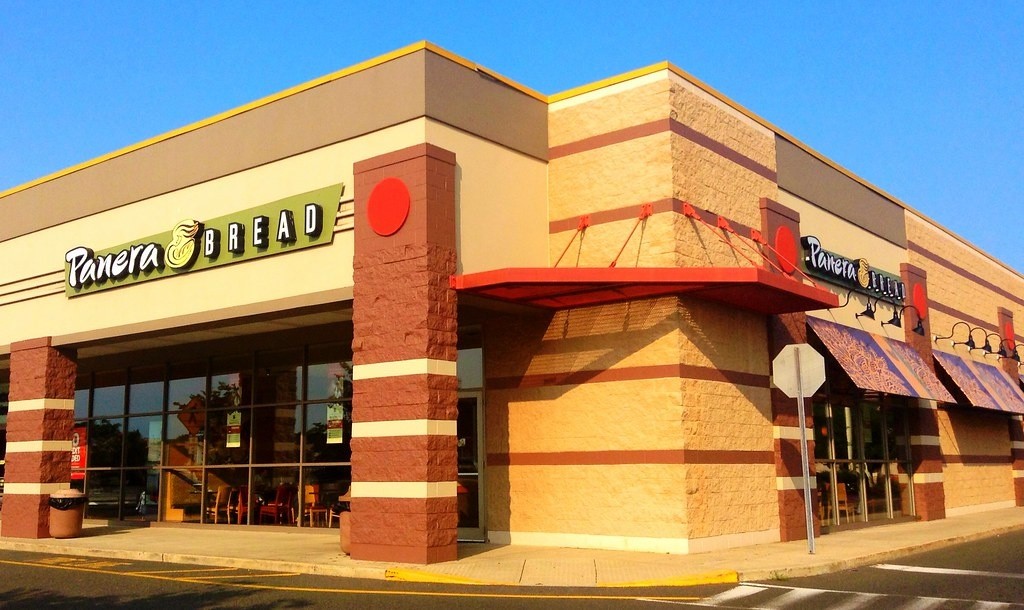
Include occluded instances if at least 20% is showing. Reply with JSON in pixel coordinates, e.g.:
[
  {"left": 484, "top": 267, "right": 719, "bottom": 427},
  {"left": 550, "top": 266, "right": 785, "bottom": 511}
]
[
  {"left": 49, "top": 488, "right": 87, "bottom": 539},
  {"left": 338, "top": 486, "right": 352, "bottom": 554}
]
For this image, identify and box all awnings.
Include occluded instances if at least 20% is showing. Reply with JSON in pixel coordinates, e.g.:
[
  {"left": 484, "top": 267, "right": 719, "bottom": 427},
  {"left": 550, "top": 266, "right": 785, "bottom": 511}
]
[
  {"left": 932, "top": 350, "right": 1024, "bottom": 415},
  {"left": 805, "top": 314, "right": 958, "bottom": 404}
]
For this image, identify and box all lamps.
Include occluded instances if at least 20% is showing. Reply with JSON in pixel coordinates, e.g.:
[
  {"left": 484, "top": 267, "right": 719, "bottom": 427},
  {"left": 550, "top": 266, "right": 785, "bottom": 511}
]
[{"left": 826, "top": 286, "right": 1024, "bottom": 366}]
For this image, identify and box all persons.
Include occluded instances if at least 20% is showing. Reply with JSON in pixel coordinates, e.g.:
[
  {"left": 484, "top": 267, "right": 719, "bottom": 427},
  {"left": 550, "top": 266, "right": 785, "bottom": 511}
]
[{"left": 815, "top": 457, "right": 902, "bottom": 519}]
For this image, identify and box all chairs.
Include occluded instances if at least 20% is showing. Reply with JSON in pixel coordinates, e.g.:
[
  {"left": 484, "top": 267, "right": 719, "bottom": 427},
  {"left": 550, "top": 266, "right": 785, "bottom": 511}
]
[
  {"left": 206, "top": 479, "right": 351, "bottom": 527},
  {"left": 825, "top": 483, "right": 855, "bottom": 525}
]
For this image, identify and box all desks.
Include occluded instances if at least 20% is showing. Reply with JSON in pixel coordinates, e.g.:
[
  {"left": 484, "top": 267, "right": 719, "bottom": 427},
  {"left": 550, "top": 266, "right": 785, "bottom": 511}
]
[{"left": 191, "top": 491, "right": 218, "bottom": 518}]
